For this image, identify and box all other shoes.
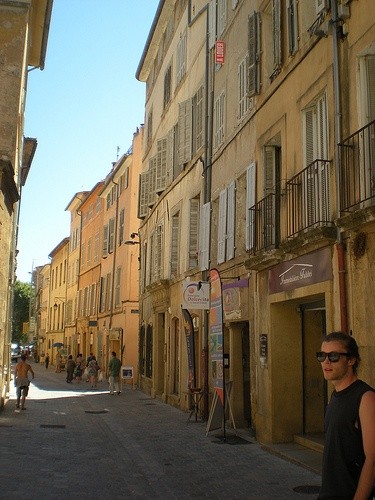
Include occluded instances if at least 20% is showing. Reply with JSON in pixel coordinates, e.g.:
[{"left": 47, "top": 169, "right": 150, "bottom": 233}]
[
  {"left": 16, "top": 402, "right": 19, "bottom": 408},
  {"left": 118, "top": 392, "right": 121, "bottom": 396},
  {"left": 22, "top": 408, "right": 26, "bottom": 410},
  {"left": 110, "top": 394, "right": 114, "bottom": 396}
]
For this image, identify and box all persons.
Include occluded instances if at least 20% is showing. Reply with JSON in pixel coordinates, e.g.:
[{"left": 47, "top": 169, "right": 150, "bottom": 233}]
[
  {"left": 316, "top": 332, "right": 375, "bottom": 500},
  {"left": 14, "top": 355, "right": 34, "bottom": 410},
  {"left": 108, "top": 352, "right": 121, "bottom": 395},
  {"left": 33, "top": 349, "right": 49, "bottom": 368},
  {"left": 66, "top": 352, "right": 100, "bottom": 387},
  {"left": 54, "top": 352, "right": 62, "bottom": 373}
]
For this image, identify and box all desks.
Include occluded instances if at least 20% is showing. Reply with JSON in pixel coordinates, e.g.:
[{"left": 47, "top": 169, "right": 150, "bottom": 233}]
[{"left": 182, "top": 391, "right": 211, "bottom": 425}]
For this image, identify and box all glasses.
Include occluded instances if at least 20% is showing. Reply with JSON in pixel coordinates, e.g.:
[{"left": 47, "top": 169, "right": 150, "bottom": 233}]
[{"left": 316, "top": 351, "right": 348, "bottom": 362}]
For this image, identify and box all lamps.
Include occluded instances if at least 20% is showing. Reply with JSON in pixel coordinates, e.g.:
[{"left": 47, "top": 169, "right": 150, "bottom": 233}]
[
  {"left": 125, "top": 241, "right": 139, "bottom": 245},
  {"left": 53, "top": 297, "right": 67, "bottom": 311},
  {"left": 38, "top": 307, "right": 47, "bottom": 317},
  {"left": 197, "top": 282, "right": 207, "bottom": 291}
]
